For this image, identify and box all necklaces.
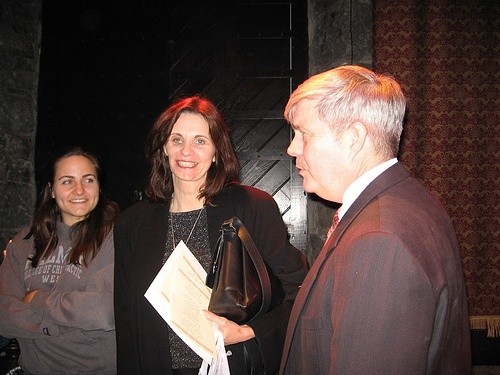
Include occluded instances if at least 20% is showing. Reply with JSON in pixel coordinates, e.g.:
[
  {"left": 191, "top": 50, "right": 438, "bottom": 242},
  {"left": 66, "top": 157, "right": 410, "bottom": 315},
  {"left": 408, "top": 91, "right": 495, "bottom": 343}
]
[{"left": 170, "top": 190, "right": 203, "bottom": 251}]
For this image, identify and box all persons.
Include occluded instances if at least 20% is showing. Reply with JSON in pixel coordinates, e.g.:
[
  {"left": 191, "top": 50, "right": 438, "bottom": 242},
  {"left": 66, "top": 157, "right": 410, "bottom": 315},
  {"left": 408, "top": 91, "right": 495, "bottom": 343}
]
[
  {"left": 0, "top": 145, "right": 118, "bottom": 375},
  {"left": 112, "top": 95, "right": 308, "bottom": 375},
  {"left": 278, "top": 64, "right": 472, "bottom": 375}
]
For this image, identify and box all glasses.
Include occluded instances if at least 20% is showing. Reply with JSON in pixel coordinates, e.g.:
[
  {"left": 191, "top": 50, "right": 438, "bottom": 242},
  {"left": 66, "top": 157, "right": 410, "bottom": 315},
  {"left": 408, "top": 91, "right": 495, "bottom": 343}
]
[{"left": 322, "top": 209, "right": 340, "bottom": 245}]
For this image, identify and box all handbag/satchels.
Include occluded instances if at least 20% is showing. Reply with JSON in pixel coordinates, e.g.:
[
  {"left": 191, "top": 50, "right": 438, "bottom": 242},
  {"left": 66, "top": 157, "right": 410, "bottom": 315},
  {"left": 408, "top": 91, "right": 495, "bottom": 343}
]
[
  {"left": 204, "top": 216, "right": 279, "bottom": 324},
  {"left": 0, "top": 337, "right": 25, "bottom": 375}
]
